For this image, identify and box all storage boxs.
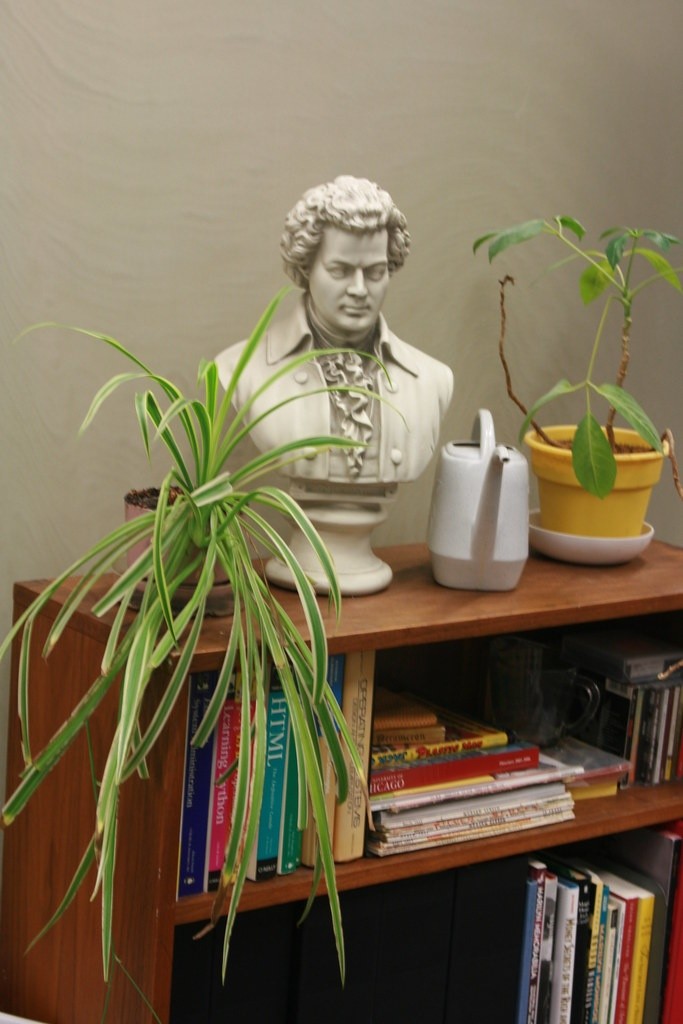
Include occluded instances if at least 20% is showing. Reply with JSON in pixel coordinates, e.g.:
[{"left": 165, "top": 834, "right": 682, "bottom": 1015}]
[{"left": 560, "top": 632, "right": 683, "bottom": 684}]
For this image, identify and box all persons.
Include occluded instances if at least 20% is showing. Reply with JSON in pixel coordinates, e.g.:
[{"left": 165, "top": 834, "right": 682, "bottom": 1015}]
[{"left": 209, "top": 174, "right": 458, "bottom": 600}]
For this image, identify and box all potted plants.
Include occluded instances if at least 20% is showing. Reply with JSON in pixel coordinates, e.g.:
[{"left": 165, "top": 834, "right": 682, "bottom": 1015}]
[
  {"left": 473, "top": 214, "right": 683, "bottom": 538},
  {"left": 0, "top": 287, "right": 408, "bottom": 1024}
]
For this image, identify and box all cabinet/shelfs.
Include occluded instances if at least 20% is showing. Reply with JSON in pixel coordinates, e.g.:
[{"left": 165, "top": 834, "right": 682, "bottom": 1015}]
[{"left": 0, "top": 540, "right": 683, "bottom": 1024}]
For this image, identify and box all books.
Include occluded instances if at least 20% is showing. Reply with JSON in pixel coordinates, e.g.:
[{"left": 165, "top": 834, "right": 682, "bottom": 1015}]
[
  {"left": 449, "top": 824, "right": 681, "bottom": 1024},
  {"left": 178, "top": 643, "right": 682, "bottom": 895}
]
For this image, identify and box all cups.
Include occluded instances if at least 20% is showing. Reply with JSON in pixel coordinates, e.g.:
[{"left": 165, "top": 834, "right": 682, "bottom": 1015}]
[{"left": 501, "top": 649, "right": 600, "bottom": 747}]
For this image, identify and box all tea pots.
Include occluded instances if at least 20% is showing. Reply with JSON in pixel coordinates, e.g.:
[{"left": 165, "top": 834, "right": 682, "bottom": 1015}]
[{"left": 428, "top": 409, "right": 529, "bottom": 589}]
